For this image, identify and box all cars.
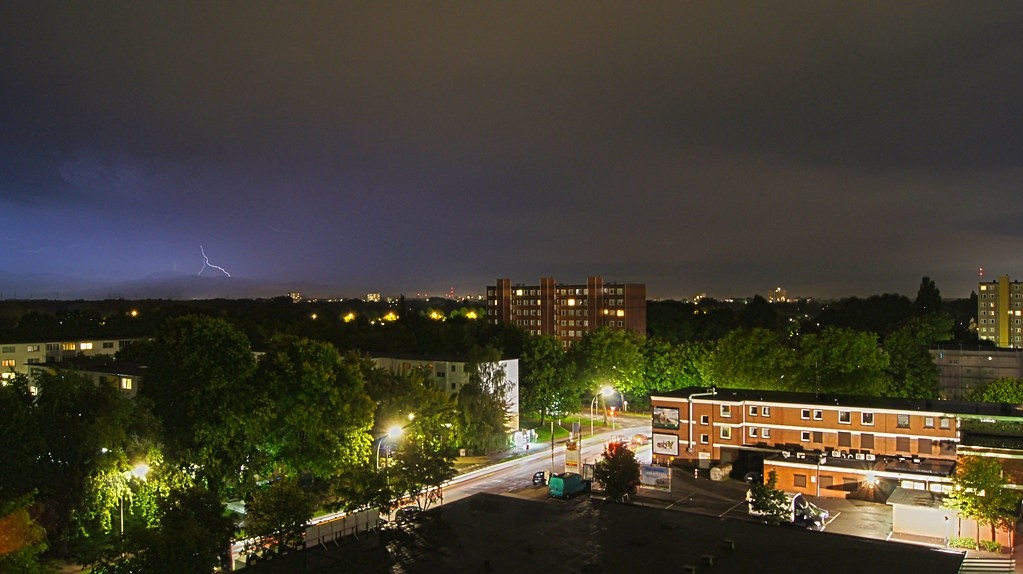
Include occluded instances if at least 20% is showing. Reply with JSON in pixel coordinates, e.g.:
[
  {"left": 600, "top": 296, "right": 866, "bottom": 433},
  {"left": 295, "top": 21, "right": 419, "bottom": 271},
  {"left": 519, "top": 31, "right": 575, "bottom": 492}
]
[
  {"left": 609, "top": 433, "right": 649, "bottom": 446},
  {"left": 743, "top": 471, "right": 763, "bottom": 483}
]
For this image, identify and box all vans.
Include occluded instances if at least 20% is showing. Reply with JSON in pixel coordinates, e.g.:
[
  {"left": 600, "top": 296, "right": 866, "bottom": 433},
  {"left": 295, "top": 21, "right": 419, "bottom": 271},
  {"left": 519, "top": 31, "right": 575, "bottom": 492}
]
[{"left": 549, "top": 472, "right": 588, "bottom": 499}]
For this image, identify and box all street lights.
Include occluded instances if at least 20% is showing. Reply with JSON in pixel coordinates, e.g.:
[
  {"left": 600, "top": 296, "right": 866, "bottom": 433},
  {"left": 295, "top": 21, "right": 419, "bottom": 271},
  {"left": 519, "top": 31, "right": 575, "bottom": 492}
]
[
  {"left": 591, "top": 387, "right": 614, "bottom": 437},
  {"left": 376, "top": 426, "right": 409, "bottom": 490},
  {"left": 121, "top": 464, "right": 150, "bottom": 561}
]
